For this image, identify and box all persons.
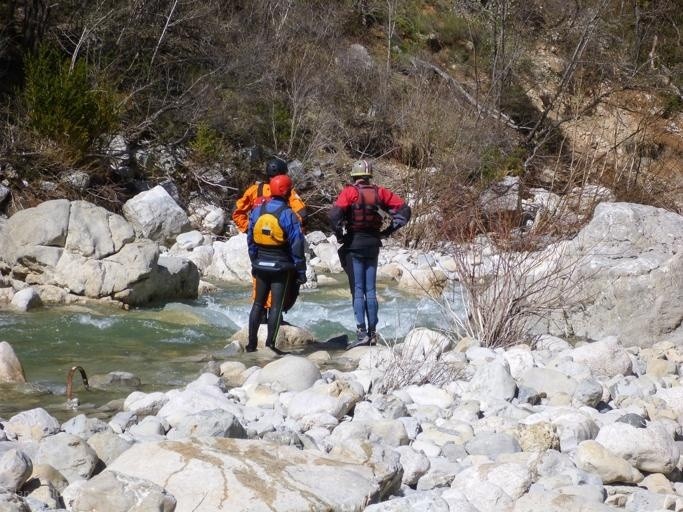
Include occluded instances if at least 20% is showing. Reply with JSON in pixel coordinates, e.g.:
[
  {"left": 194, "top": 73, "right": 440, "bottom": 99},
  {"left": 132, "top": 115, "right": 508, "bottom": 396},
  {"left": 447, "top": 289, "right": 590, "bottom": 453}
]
[
  {"left": 328, "top": 160, "right": 410, "bottom": 350},
  {"left": 246, "top": 176, "right": 307, "bottom": 354},
  {"left": 232, "top": 159, "right": 308, "bottom": 325}
]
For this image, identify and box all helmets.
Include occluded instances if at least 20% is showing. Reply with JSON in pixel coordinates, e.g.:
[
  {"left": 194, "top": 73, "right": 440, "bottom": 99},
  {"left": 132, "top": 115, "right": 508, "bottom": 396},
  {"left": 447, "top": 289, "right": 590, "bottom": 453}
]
[
  {"left": 351, "top": 160, "right": 373, "bottom": 178},
  {"left": 270, "top": 175, "right": 292, "bottom": 198},
  {"left": 267, "top": 159, "right": 288, "bottom": 176}
]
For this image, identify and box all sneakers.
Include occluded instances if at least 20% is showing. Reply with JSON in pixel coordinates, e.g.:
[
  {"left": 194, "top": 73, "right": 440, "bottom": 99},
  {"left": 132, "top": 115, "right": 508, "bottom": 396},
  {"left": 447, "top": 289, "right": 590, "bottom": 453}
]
[
  {"left": 262, "top": 310, "right": 288, "bottom": 325},
  {"left": 345, "top": 332, "right": 369, "bottom": 350},
  {"left": 368, "top": 333, "right": 377, "bottom": 345}
]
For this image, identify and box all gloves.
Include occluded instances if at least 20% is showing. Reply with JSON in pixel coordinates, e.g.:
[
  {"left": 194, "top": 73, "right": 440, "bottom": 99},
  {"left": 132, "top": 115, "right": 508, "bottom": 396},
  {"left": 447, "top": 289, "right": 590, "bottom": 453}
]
[
  {"left": 252, "top": 268, "right": 256, "bottom": 278},
  {"left": 296, "top": 274, "right": 307, "bottom": 285},
  {"left": 380, "top": 226, "right": 390, "bottom": 238},
  {"left": 335, "top": 230, "right": 344, "bottom": 243}
]
